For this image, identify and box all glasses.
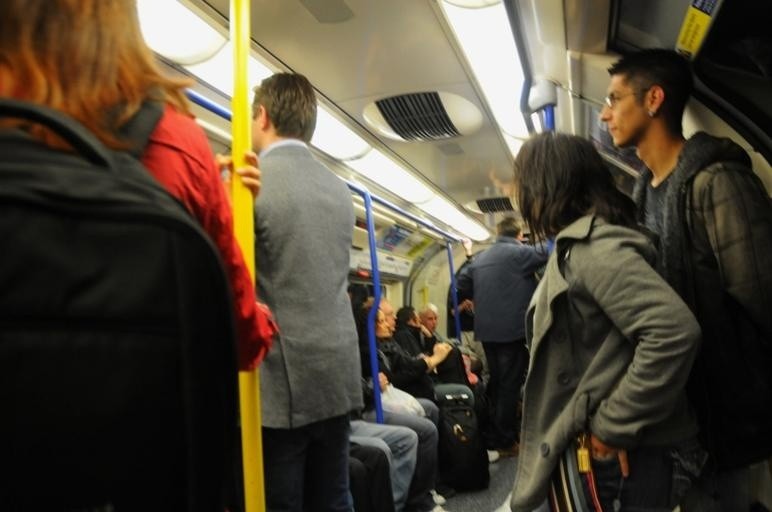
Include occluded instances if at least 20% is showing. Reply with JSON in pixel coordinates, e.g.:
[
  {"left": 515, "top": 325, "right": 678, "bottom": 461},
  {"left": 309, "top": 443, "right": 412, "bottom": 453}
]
[{"left": 605, "top": 92, "right": 642, "bottom": 108}]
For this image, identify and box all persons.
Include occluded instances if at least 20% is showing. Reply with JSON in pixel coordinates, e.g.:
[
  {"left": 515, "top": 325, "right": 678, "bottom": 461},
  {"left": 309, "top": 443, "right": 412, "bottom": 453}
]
[
  {"left": 598, "top": 47, "right": 772, "bottom": 512},
  {"left": 345, "top": 285, "right": 531, "bottom": 512},
  {"left": 0, "top": 0, "right": 281, "bottom": 511},
  {"left": 454, "top": 217, "right": 548, "bottom": 457},
  {"left": 510, "top": 128, "right": 703, "bottom": 512},
  {"left": 253, "top": 73, "right": 367, "bottom": 512}
]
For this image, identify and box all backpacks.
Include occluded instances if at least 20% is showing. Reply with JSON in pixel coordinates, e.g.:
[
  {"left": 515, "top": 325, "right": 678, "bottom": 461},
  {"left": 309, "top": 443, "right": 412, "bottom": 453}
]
[{"left": 1, "top": 86, "right": 245, "bottom": 512}]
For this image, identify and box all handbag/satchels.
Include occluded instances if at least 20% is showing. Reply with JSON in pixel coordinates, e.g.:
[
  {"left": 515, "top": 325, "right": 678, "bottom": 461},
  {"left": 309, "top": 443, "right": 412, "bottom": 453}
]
[{"left": 436, "top": 342, "right": 487, "bottom": 416}]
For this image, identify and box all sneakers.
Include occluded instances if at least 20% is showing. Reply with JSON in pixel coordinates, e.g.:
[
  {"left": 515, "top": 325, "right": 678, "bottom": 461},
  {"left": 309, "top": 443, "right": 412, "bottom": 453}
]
[
  {"left": 429, "top": 490, "right": 446, "bottom": 504},
  {"left": 431, "top": 505, "right": 447, "bottom": 512},
  {"left": 493, "top": 442, "right": 520, "bottom": 457},
  {"left": 487, "top": 450, "right": 499, "bottom": 462}
]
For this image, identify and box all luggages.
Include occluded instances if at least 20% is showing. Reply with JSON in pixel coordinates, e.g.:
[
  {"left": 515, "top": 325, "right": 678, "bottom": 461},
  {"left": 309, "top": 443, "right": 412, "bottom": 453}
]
[{"left": 434, "top": 394, "right": 489, "bottom": 492}]
